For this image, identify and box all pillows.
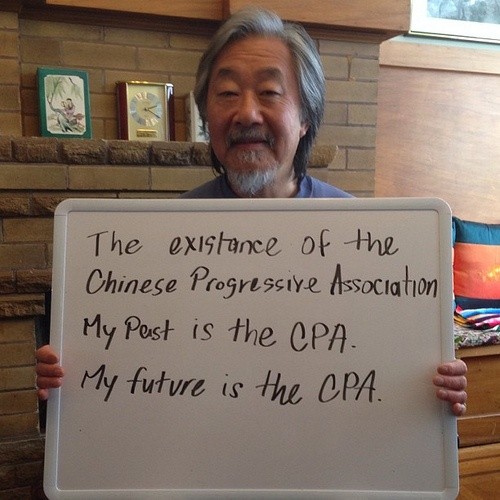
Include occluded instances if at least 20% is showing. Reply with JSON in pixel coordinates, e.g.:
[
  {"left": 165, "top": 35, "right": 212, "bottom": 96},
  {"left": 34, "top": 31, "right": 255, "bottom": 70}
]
[{"left": 452, "top": 216, "right": 500, "bottom": 310}]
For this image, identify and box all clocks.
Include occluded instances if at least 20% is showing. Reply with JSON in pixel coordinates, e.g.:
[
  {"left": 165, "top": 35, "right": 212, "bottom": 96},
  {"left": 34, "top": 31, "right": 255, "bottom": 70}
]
[{"left": 116, "top": 81, "right": 176, "bottom": 142}]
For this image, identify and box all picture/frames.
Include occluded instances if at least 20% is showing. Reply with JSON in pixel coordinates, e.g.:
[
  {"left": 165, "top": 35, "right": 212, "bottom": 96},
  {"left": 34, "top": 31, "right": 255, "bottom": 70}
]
[
  {"left": 38, "top": 67, "right": 92, "bottom": 139},
  {"left": 404, "top": 0, "right": 500, "bottom": 45},
  {"left": 185, "top": 91, "right": 211, "bottom": 143}
]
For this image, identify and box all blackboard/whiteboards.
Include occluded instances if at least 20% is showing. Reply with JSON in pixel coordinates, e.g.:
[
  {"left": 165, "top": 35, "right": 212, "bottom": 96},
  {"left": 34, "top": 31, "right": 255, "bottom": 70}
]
[{"left": 42, "top": 198, "right": 461, "bottom": 500}]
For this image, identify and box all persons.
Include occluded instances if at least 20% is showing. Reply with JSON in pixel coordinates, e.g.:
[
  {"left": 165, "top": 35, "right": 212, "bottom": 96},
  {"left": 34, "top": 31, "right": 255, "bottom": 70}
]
[{"left": 34, "top": 7, "right": 467, "bottom": 416}]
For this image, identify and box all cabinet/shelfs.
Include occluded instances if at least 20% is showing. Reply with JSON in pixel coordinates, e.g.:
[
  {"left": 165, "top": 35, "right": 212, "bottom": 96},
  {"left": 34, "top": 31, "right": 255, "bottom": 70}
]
[{"left": 454, "top": 323, "right": 500, "bottom": 448}]
